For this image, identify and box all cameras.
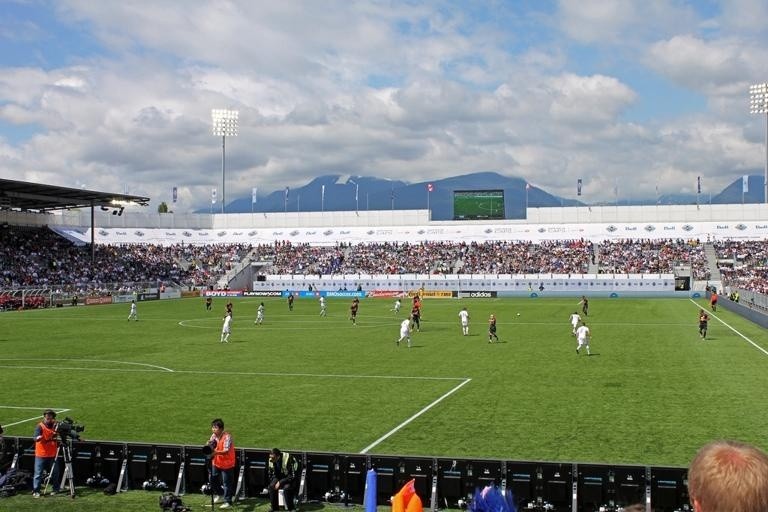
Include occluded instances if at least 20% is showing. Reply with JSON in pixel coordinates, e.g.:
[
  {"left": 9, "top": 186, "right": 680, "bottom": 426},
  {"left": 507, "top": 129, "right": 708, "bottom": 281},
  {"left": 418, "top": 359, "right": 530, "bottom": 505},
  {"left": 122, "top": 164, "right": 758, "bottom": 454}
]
[{"left": 202, "top": 440, "right": 217, "bottom": 453}]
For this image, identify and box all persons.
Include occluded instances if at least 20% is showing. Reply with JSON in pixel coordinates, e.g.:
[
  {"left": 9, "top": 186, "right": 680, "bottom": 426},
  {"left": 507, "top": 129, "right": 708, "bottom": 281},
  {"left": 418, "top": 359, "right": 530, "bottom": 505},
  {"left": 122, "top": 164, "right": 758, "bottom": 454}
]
[
  {"left": 1, "top": 224, "right": 768, "bottom": 311},
  {"left": 570, "top": 296, "right": 591, "bottom": 355},
  {"left": 32, "top": 409, "right": 84, "bottom": 498},
  {"left": 205, "top": 419, "right": 237, "bottom": 508},
  {"left": 268, "top": 447, "right": 302, "bottom": 512},
  {"left": 0, "top": 433, "right": 30, "bottom": 498},
  {"left": 396, "top": 307, "right": 420, "bottom": 347},
  {"left": 128, "top": 300, "right": 138, "bottom": 321},
  {"left": 685, "top": 440, "right": 768, "bottom": 512},
  {"left": 699, "top": 308, "right": 710, "bottom": 339},
  {"left": 350, "top": 300, "right": 358, "bottom": 325},
  {"left": 459, "top": 308, "right": 499, "bottom": 344},
  {"left": 220, "top": 301, "right": 264, "bottom": 343}
]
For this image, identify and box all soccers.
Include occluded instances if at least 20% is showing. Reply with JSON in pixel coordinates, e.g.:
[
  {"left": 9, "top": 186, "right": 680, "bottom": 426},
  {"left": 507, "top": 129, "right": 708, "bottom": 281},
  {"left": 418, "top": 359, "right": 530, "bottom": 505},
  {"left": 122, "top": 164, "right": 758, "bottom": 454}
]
[{"left": 517, "top": 313, "right": 520, "bottom": 316}]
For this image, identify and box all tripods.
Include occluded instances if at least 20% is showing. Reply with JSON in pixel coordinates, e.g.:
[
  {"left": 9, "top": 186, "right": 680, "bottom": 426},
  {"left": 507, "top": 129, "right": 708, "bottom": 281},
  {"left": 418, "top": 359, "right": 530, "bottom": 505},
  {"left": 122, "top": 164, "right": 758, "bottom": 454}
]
[{"left": 42, "top": 440, "right": 76, "bottom": 499}]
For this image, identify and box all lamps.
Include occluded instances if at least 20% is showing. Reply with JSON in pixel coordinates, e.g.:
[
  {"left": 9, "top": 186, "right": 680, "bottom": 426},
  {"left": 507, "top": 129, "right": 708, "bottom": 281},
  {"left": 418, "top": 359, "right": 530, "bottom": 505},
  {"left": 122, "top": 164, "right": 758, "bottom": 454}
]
[
  {"left": 117, "top": 206, "right": 124, "bottom": 216},
  {"left": 112, "top": 206, "right": 117, "bottom": 215},
  {"left": 100, "top": 207, "right": 108, "bottom": 212}
]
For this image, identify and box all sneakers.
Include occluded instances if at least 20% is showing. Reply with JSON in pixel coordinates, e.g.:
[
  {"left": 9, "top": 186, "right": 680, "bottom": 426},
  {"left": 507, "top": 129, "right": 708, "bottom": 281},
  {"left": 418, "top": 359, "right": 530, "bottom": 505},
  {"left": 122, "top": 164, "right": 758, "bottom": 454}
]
[
  {"left": 220, "top": 503, "right": 232, "bottom": 509},
  {"left": 214, "top": 495, "right": 221, "bottom": 503},
  {"left": 33, "top": 491, "right": 40, "bottom": 499}
]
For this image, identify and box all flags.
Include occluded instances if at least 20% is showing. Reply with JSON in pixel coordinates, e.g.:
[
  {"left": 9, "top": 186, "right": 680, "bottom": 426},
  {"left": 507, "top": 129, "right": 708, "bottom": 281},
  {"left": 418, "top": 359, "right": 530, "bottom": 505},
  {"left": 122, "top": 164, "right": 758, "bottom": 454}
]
[{"left": 123, "top": 175, "right": 746, "bottom": 206}]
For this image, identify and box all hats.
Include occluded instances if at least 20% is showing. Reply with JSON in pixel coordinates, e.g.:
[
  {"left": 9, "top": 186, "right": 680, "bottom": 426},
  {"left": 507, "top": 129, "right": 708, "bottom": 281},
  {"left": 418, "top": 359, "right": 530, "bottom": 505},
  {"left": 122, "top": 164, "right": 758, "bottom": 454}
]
[{"left": 43, "top": 409, "right": 57, "bottom": 418}]
